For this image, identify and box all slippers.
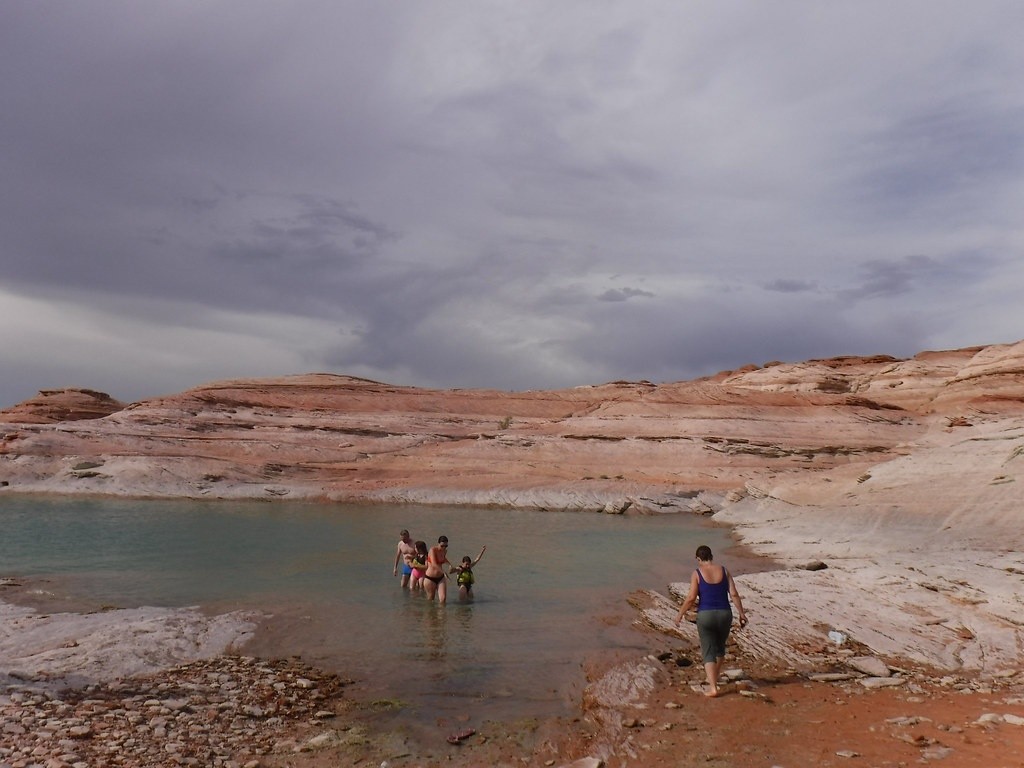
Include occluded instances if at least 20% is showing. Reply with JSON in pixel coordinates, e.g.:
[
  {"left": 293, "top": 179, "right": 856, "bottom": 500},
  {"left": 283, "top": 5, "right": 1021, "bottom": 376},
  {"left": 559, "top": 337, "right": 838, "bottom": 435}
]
[
  {"left": 717, "top": 674, "right": 731, "bottom": 686},
  {"left": 699, "top": 681, "right": 712, "bottom": 692}
]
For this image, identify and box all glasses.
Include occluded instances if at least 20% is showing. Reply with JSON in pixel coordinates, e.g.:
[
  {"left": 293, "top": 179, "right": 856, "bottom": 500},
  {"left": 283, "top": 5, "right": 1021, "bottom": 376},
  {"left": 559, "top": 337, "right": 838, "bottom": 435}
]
[{"left": 439, "top": 543, "right": 448, "bottom": 548}]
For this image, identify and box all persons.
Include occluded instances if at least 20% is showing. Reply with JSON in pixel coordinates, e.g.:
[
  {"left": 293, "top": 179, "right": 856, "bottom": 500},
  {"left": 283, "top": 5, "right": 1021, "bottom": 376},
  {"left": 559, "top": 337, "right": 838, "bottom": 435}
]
[
  {"left": 424, "top": 536, "right": 452, "bottom": 604},
  {"left": 393, "top": 530, "right": 417, "bottom": 588},
  {"left": 410, "top": 541, "right": 428, "bottom": 590},
  {"left": 675, "top": 545, "right": 748, "bottom": 696},
  {"left": 448, "top": 544, "right": 485, "bottom": 600}
]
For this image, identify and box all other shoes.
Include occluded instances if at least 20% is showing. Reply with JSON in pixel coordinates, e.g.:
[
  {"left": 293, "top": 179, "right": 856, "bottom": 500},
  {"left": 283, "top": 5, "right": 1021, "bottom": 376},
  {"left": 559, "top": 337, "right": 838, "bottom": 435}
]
[
  {"left": 676, "top": 658, "right": 692, "bottom": 667},
  {"left": 658, "top": 652, "right": 671, "bottom": 660}
]
